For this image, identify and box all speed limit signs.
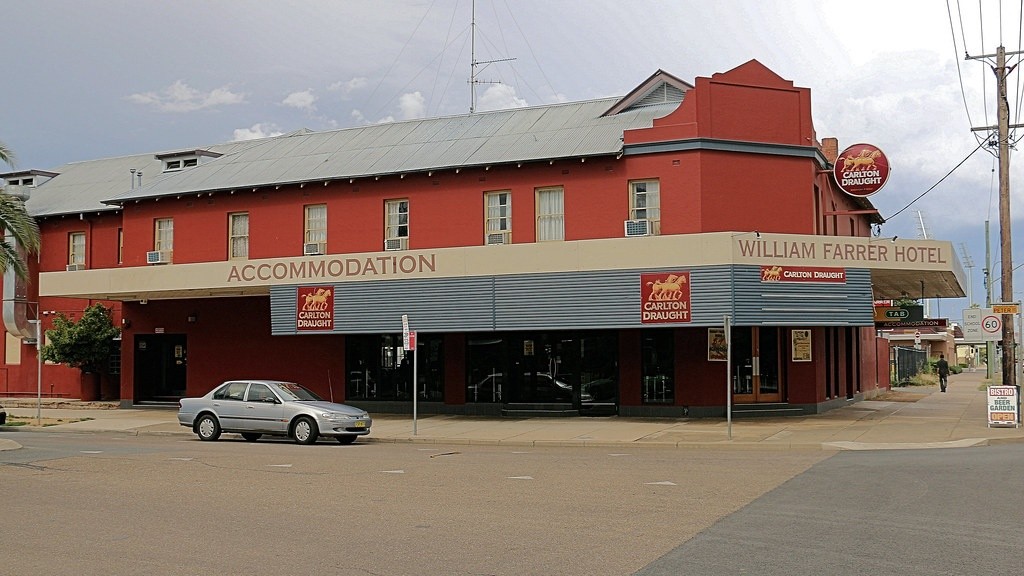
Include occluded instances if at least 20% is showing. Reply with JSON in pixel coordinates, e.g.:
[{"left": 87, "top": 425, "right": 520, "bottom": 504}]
[{"left": 982, "top": 316, "right": 1001, "bottom": 333}]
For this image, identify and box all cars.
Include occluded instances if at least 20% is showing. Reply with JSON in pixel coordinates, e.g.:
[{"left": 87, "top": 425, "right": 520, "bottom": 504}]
[
  {"left": 175, "top": 379, "right": 373, "bottom": 444},
  {"left": 467, "top": 371, "right": 574, "bottom": 403}
]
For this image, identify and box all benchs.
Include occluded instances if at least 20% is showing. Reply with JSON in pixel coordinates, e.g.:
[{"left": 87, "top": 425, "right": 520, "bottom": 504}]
[{"left": 230, "top": 391, "right": 259, "bottom": 400}]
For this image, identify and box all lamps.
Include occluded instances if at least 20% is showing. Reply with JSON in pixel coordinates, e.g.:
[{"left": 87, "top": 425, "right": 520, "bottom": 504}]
[
  {"left": 869, "top": 236, "right": 898, "bottom": 243},
  {"left": 732, "top": 230, "right": 761, "bottom": 238}
]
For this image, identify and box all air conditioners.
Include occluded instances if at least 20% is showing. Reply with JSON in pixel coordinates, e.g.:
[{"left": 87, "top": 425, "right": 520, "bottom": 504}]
[
  {"left": 624, "top": 218, "right": 657, "bottom": 237},
  {"left": 146, "top": 251, "right": 170, "bottom": 264},
  {"left": 66, "top": 264, "right": 85, "bottom": 271},
  {"left": 384, "top": 238, "right": 407, "bottom": 251},
  {"left": 485, "top": 232, "right": 508, "bottom": 244},
  {"left": 304, "top": 242, "right": 324, "bottom": 255}
]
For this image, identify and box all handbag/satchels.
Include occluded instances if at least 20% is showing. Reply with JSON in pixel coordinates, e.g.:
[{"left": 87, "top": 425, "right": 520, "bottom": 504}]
[{"left": 940, "top": 361, "right": 949, "bottom": 374}]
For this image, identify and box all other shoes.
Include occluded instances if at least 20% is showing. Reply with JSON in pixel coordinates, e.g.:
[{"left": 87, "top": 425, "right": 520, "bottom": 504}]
[
  {"left": 945, "top": 386, "right": 946, "bottom": 388},
  {"left": 941, "top": 391, "right": 945, "bottom": 392}
]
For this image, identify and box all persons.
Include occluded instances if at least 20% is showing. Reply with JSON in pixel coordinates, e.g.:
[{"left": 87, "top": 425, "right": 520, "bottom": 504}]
[
  {"left": 392, "top": 359, "right": 412, "bottom": 376},
  {"left": 930, "top": 355, "right": 951, "bottom": 394}
]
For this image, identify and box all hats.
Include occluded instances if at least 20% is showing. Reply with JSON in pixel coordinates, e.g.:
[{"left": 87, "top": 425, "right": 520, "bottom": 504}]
[{"left": 940, "top": 354, "right": 944, "bottom": 358}]
